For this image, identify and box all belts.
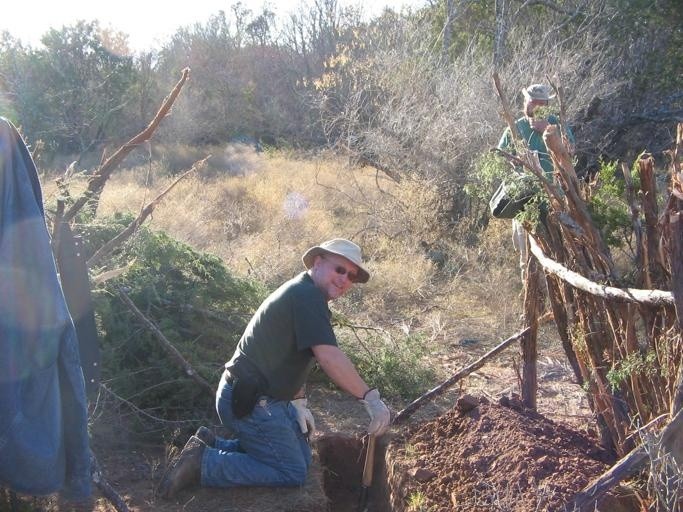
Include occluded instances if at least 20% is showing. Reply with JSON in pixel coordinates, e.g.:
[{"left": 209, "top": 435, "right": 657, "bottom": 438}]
[{"left": 222, "top": 369, "right": 233, "bottom": 385}]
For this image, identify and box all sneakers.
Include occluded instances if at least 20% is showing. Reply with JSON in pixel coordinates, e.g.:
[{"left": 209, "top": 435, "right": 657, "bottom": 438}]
[{"left": 156, "top": 425, "right": 216, "bottom": 499}]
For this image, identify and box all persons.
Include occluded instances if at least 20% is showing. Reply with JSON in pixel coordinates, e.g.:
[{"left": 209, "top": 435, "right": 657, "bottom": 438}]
[
  {"left": 494, "top": 83, "right": 576, "bottom": 319},
  {"left": 154, "top": 237, "right": 391, "bottom": 500}
]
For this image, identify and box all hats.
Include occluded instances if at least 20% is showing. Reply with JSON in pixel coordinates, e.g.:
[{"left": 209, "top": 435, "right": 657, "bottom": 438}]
[
  {"left": 302, "top": 237, "right": 370, "bottom": 284},
  {"left": 521, "top": 83, "right": 556, "bottom": 101}
]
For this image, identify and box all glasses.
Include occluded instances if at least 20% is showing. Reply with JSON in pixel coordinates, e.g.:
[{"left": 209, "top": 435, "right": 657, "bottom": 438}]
[{"left": 321, "top": 256, "right": 357, "bottom": 282}]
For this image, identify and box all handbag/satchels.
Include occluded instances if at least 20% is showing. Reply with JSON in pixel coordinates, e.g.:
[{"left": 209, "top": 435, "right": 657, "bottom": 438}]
[{"left": 488, "top": 174, "right": 538, "bottom": 218}]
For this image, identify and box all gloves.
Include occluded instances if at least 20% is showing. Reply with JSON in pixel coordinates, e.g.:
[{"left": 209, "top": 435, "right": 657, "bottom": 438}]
[
  {"left": 290, "top": 397, "right": 314, "bottom": 439},
  {"left": 356, "top": 386, "right": 391, "bottom": 439}
]
